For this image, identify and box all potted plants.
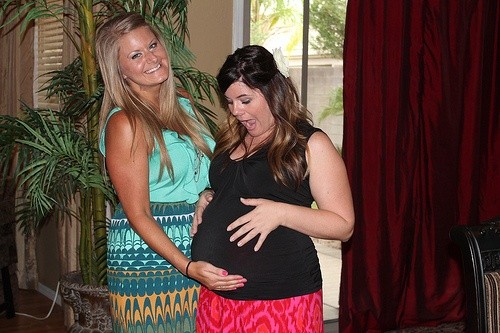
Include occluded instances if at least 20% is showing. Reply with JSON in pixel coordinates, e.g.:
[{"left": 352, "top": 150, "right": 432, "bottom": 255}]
[{"left": 0, "top": 0, "right": 219, "bottom": 333}]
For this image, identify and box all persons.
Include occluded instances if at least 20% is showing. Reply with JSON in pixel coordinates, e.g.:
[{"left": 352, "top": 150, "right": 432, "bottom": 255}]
[
  {"left": 94, "top": 12, "right": 247, "bottom": 333},
  {"left": 190, "top": 45, "right": 355, "bottom": 333}
]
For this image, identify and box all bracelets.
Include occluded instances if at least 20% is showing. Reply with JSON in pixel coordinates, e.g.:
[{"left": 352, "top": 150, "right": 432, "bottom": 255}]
[{"left": 186, "top": 261, "right": 192, "bottom": 278}]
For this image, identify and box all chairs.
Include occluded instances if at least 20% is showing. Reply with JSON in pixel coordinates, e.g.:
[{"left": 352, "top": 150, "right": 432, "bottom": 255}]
[{"left": 449, "top": 215, "right": 500, "bottom": 333}]
